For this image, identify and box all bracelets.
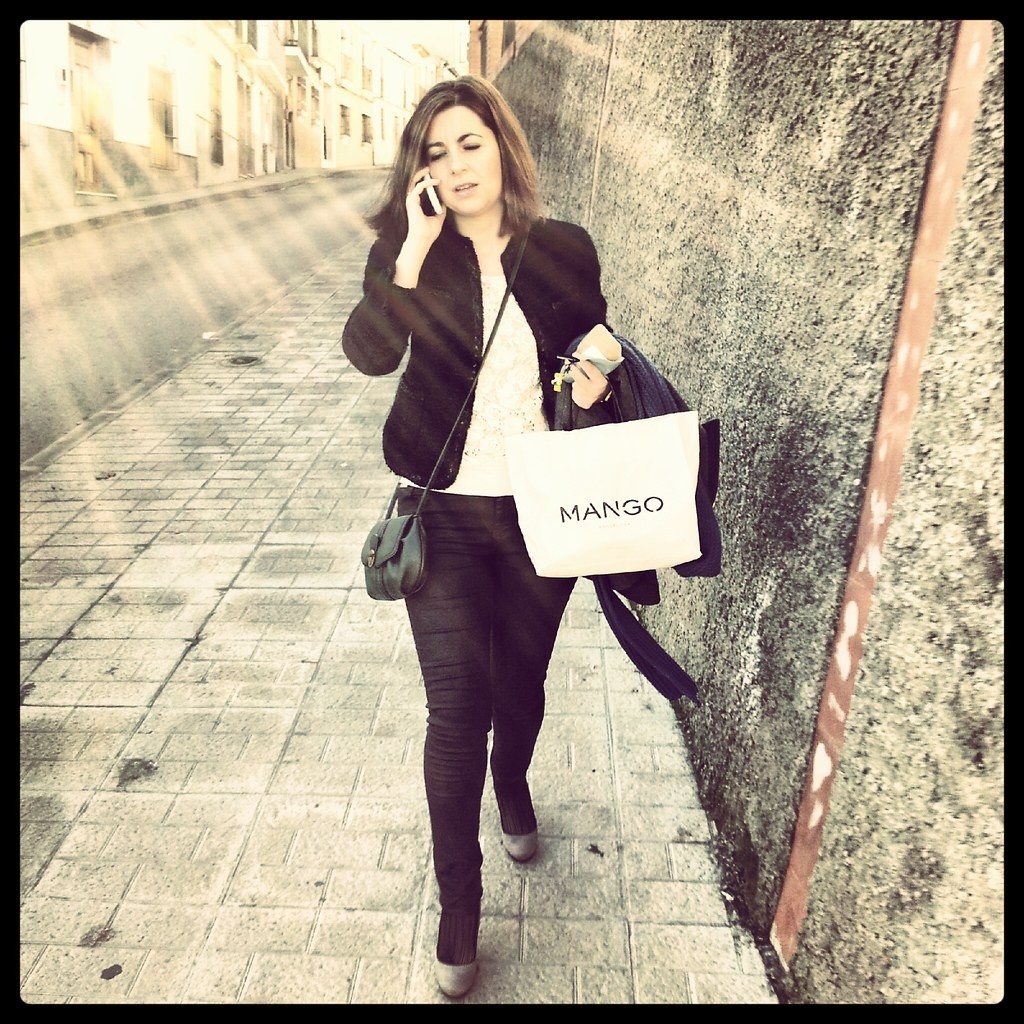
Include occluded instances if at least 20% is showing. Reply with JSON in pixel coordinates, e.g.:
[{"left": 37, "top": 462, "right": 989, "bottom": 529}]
[{"left": 600, "top": 377, "right": 615, "bottom": 404}]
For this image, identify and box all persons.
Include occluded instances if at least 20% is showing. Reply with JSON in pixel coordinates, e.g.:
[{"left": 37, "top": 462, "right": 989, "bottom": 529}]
[{"left": 342, "top": 73, "right": 628, "bottom": 998}]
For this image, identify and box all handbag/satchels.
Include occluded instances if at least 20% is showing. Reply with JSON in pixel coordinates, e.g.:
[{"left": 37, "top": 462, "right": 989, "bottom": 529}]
[
  {"left": 504, "top": 374, "right": 702, "bottom": 578},
  {"left": 361, "top": 514, "right": 430, "bottom": 601}
]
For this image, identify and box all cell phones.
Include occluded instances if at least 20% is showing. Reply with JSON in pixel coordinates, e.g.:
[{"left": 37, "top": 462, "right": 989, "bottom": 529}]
[{"left": 424, "top": 172, "right": 443, "bottom": 216}]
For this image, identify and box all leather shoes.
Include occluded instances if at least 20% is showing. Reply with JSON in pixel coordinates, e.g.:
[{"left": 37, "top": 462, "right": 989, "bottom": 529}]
[
  {"left": 489, "top": 754, "right": 537, "bottom": 862},
  {"left": 434, "top": 886, "right": 484, "bottom": 998}
]
